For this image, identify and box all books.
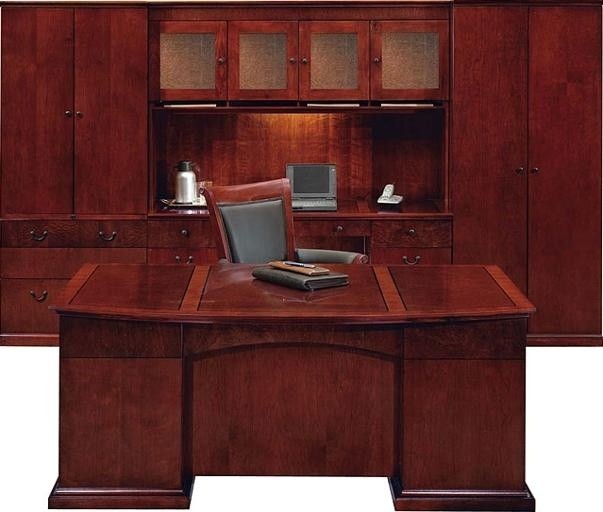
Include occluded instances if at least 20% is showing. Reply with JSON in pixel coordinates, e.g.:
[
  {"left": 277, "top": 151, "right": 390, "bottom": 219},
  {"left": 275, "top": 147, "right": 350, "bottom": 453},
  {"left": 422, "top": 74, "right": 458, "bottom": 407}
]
[{"left": 252, "top": 261, "right": 350, "bottom": 291}]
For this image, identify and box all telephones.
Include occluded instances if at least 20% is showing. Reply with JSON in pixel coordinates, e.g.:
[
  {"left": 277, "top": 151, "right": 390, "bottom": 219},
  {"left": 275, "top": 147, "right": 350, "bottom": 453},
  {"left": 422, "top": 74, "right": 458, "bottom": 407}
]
[{"left": 377, "top": 184, "right": 403, "bottom": 208}]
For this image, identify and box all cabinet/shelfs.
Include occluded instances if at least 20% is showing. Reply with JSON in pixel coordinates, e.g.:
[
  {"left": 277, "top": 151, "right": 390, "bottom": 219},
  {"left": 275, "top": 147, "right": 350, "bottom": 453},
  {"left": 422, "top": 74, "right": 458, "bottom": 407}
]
[
  {"left": 443, "top": 2, "right": 600, "bottom": 347},
  {"left": 0, "top": 216, "right": 145, "bottom": 346},
  {"left": 227, "top": 0, "right": 369, "bottom": 100},
  {"left": 1, "top": 1, "right": 166, "bottom": 218},
  {"left": 366, "top": 0, "right": 448, "bottom": 103},
  {"left": 146, "top": 219, "right": 452, "bottom": 267},
  {"left": 147, "top": 0, "right": 226, "bottom": 99}
]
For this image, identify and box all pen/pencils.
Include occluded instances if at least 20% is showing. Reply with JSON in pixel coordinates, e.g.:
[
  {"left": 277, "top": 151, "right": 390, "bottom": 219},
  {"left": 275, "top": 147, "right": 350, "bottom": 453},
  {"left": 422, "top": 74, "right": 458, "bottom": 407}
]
[{"left": 285, "top": 262, "right": 315, "bottom": 268}]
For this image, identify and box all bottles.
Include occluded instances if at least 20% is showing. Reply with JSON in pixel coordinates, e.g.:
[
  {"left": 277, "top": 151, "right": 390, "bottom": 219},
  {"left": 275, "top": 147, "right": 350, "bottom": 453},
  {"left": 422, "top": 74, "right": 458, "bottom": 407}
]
[{"left": 171, "top": 160, "right": 200, "bottom": 204}]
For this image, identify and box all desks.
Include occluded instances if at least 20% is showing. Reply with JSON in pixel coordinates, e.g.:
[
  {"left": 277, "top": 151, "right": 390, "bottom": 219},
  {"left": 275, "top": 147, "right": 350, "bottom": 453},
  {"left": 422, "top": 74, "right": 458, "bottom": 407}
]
[{"left": 50, "top": 258, "right": 540, "bottom": 508}]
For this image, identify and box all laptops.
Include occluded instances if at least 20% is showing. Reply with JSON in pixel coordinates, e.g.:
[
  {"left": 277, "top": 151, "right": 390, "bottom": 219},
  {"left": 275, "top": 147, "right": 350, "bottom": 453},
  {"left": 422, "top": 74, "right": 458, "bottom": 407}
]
[{"left": 286, "top": 162, "right": 337, "bottom": 211}]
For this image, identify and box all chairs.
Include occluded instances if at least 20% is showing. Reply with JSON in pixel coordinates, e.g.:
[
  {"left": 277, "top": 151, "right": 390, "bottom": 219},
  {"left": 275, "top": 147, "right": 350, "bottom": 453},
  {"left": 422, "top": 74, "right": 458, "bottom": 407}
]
[{"left": 202, "top": 178, "right": 369, "bottom": 266}]
